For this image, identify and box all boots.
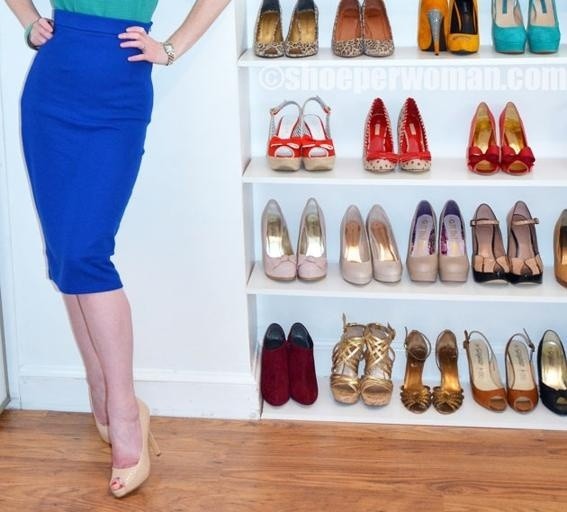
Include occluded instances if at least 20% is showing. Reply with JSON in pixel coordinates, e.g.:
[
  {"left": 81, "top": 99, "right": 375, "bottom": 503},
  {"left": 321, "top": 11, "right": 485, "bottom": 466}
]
[
  {"left": 261, "top": 323, "right": 289, "bottom": 406},
  {"left": 288, "top": 323, "right": 318, "bottom": 405}
]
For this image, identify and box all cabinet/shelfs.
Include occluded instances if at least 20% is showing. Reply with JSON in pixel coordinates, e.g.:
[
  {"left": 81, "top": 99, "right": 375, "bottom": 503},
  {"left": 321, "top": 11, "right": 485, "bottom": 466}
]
[{"left": 229, "top": 43, "right": 567, "bottom": 432}]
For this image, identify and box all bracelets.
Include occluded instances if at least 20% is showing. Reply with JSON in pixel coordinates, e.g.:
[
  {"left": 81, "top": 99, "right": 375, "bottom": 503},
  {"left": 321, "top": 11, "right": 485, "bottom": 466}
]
[
  {"left": 162, "top": 41, "right": 175, "bottom": 68},
  {"left": 23, "top": 20, "right": 37, "bottom": 52}
]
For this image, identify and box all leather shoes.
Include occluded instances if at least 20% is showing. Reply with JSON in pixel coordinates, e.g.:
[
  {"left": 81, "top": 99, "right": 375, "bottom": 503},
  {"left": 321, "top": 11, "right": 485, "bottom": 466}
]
[
  {"left": 525, "top": 1, "right": 560, "bottom": 53},
  {"left": 406, "top": 200, "right": 438, "bottom": 283},
  {"left": 332, "top": 1, "right": 363, "bottom": 57},
  {"left": 366, "top": 204, "right": 403, "bottom": 283},
  {"left": 397, "top": 97, "right": 432, "bottom": 172},
  {"left": 434, "top": 200, "right": 469, "bottom": 281},
  {"left": 363, "top": 97, "right": 397, "bottom": 171},
  {"left": 553, "top": 208, "right": 567, "bottom": 287},
  {"left": 363, "top": 0, "right": 395, "bottom": 57},
  {"left": 297, "top": 198, "right": 327, "bottom": 281},
  {"left": 340, "top": 204, "right": 372, "bottom": 285},
  {"left": 491, "top": 1, "right": 528, "bottom": 53},
  {"left": 261, "top": 199, "right": 297, "bottom": 280}
]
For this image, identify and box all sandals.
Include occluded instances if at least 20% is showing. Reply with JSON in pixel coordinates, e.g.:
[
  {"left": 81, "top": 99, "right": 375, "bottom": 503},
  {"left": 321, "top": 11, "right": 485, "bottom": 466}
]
[
  {"left": 360, "top": 324, "right": 395, "bottom": 407},
  {"left": 464, "top": 330, "right": 508, "bottom": 411},
  {"left": 301, "top": 97, "right": 335, "bottom": 171},
  {"left": 470, "top": 204, "right": 511, "bottom": 284},
  {"left": 285, "top": 1, "right": 319, "bottom": 58},
  {"left": 267, "top": 100, "right": 302, "bottom": 172},
  {"left": 400, "top": 326, "right": 432, "bottom": 413},
  {"left": 253, "top": 1, "right": 285, "bottom": 57},
  {"left": 432, "top": 329, "right": 464, "bottom": 414},
  {"left": 537, "top": 329, "right": 567, "bottom": 416},
  {"left": 505, "top": 327, "right": 539, "bottom": 413},
  {"left": 468, "top": 102, "right": 500, "bottom": 175},
  {"left": 330, "top": 324, "right": 372, "bottom": 405},
  {"left": 507, "top": 201, "right": 543, "bottom": 285},
  {"left": 499, "top": 102, "right": 535, "bottom": 175}
]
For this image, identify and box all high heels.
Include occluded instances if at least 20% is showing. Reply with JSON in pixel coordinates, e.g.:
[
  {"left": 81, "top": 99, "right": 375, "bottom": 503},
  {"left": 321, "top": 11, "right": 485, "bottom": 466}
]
[
  {"left": 448, "top": 2, "right": 479, "bottom": 52},
  {"left": 110, "top": 395, "right": 160, "bottom": 499},
  {"left": 418, "top": 0, "right": 448, "bottom": 55},
  {"left": 88, "top": 385, "right": 112, "bottom": 442}
]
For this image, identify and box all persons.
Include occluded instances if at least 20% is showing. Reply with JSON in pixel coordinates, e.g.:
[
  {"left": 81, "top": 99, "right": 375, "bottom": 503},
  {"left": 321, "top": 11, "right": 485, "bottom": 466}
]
[{"left": 5, "top": 0, "right": 230, "bottom": 500}]
